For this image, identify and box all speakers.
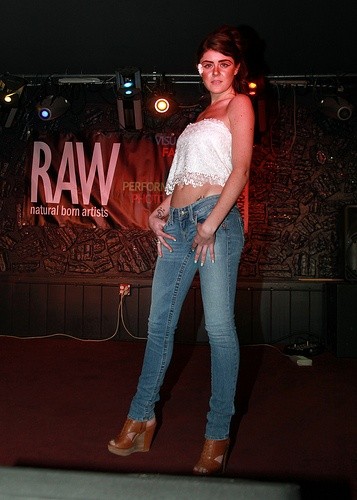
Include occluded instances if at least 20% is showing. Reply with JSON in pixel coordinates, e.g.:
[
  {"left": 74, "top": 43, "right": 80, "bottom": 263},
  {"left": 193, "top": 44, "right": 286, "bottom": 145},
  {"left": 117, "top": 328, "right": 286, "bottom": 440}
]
[
  {"left": 335, "top": 204, "right": 357, "bottom": 282},
  {"left": 326, "top": 281, "right": 357, "bottom": 358}
]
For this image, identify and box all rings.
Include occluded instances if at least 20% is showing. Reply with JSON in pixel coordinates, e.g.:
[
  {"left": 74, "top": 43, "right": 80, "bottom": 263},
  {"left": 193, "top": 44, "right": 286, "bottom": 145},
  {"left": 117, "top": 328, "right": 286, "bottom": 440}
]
[{"left": 157, "top": 241, "right": 161, "bottom": 244}]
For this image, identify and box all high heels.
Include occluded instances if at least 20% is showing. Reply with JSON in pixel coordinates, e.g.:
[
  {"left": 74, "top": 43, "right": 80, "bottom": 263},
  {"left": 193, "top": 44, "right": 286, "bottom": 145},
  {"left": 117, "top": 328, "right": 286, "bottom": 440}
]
[
  {"left": 193, "top": 437, "right": 229, "bottom": 477},
  {"left": 108, "top": 416, "right": 157, "bottom": 456}
]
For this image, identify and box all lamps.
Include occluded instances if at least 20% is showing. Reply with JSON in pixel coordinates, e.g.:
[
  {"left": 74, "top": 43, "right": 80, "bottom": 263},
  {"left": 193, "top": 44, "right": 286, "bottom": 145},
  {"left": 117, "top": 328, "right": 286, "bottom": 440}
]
[
  {"left": 153, "top": 94, "right": 174, "bottom": 114},
  {"left": 119, "top": 75, "right": 140, "bottom": 97},
  {"left": 38, "top": 95, "right": 70, "bottom": 121},
  {"left": 320, "top": 96, "right": 351, "bottom": 121},
  {"left": 5, "top": 92, "right": 19, "bottom": 103}
]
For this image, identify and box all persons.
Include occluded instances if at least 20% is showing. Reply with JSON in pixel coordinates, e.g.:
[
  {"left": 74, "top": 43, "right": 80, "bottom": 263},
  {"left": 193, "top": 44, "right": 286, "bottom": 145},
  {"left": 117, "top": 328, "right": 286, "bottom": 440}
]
[{"left": 107, "top": 25, "right": 255, "bottom": 474}]
